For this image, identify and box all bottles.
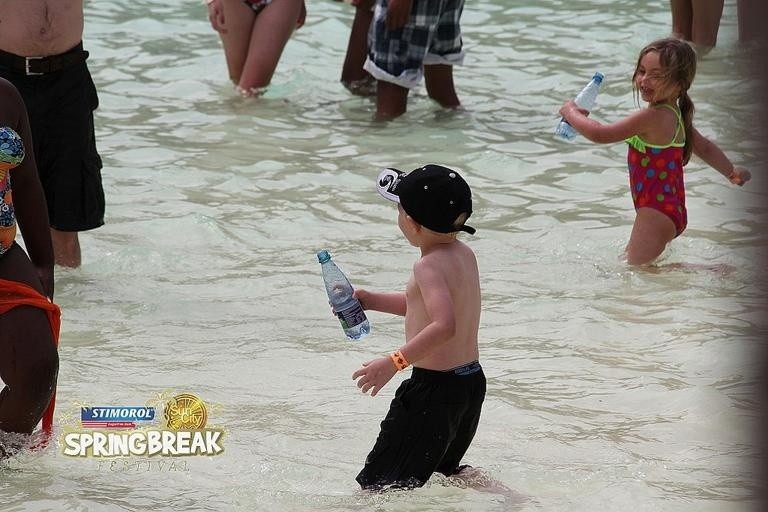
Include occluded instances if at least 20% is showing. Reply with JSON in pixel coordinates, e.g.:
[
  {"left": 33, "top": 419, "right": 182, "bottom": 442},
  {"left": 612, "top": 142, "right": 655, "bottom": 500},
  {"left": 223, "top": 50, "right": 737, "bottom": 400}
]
[
  {"left": 554, "top": 71, "right": 605, "bottom": 143},
  {"left": 316, "top": 249, "right": 371, "bottom": 340}
]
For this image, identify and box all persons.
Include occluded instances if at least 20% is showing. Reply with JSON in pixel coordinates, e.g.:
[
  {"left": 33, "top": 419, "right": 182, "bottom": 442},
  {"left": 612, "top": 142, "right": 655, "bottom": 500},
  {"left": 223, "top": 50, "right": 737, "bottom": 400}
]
[
  {"left": 670, "top": 0, "right": 724, "bottom": 48},
  {"left": 559, "top": 37, "right": 752, "bottom": 264},
  {"left": 329, "top": 165, "right": 487, "bottom": 490},
  {"left": 0, "top": 0, "right": 105, "bottom": 461},
  {"left": 206, "top": 0, "right": 465, "bottom": 119}
]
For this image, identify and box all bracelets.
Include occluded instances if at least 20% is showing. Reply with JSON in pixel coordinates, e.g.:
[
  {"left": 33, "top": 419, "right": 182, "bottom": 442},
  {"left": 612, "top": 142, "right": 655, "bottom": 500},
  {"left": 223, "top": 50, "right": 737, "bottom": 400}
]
[
  {"left": 390, "top": 350, "right": 409, "bottom": 371},
  {"left": 728, "top": 166, "right": 738, "bottom": 180}
]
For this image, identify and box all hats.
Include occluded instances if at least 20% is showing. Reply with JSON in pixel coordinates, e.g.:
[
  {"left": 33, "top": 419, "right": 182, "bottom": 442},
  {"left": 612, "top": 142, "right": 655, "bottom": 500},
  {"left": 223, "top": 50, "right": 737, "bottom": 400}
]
[{"left": 374, "top": 162, "right": 477, "bottom": 236}]
